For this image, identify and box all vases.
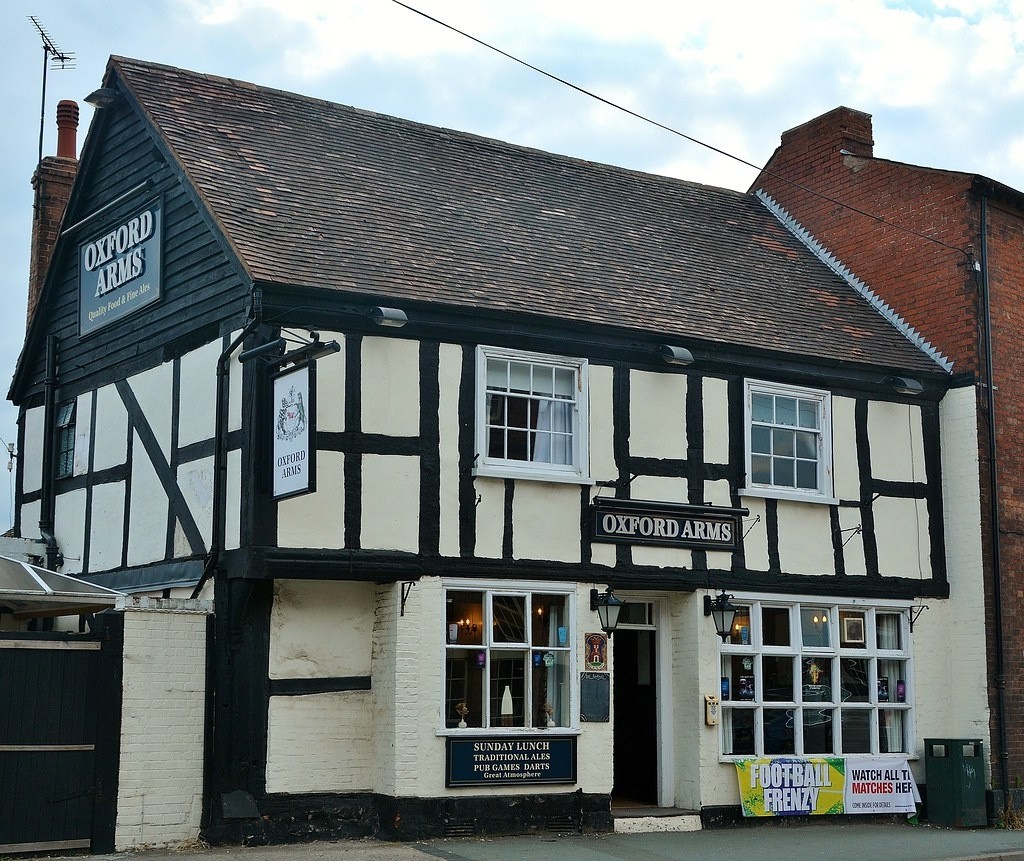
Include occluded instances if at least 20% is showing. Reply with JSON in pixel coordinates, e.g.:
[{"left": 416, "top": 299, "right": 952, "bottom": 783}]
[{"left": 811, "top": 674, "right": 819, "bottom": 684}]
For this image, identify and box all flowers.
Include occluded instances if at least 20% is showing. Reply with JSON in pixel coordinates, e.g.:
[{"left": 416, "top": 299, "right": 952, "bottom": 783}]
[{"left": 808, "top": 663, "right": 823, "bottom": 678}]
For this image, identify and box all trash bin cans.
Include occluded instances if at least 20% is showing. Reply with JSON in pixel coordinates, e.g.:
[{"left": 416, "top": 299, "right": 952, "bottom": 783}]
[{"left": 924, "top": 736, "right": 989, "bottom": 827}]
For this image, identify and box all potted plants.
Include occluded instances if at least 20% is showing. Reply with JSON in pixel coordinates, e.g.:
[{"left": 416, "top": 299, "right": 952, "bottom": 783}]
[
  {"left": 545, "top": 702, "right": 555, "bottom": 726},
  {"left": 455, "top": 703, "right": 469, "bottom": 727}
]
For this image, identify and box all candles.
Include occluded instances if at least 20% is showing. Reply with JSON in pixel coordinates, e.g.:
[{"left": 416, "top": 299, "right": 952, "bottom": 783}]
[
  {"left": 813, "top": 616, "right": 818, "bottom": 626},
  {"left": 460, "top": 619, "right": 463, "bottom": 628},
  {"left": 822, "top": 616, "right": 826, "bottom": 626},
  {"left": 466, "top": 618, "right": 470, "bottom": 628},
  {"left": 736, "top": 624, "right": 739, "bottom": 633}
]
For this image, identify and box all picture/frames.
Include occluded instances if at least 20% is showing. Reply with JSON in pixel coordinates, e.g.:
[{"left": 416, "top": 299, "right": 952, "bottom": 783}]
[{"left": 844, "top": 618, "right": 864, "bottom": 643}]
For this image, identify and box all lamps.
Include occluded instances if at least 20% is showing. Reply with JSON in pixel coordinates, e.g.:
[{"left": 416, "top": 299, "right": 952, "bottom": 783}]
[
  {"left": 704, "top": 589, "right": 740, "bottom": 642},
  {"left": 538, "top": 608, "right": 543, "bottom": 618},
  {"left": 83, "top": 88, "right": 121, "bottom": 109},
  {"left": 658, "top": 345, "right": 694, "bottom": 366},
  {"left": 891, "top": 376, "right": 924, "bottom": 395},
  {"left": 6, "top": 443, "right": 17, "bottom": 473},
  {"left": 590, "top": 586, "right": 626, "bottom": 639},
  {"left": 366, "top": 306, "right": 411, "bottom": 328}
]
